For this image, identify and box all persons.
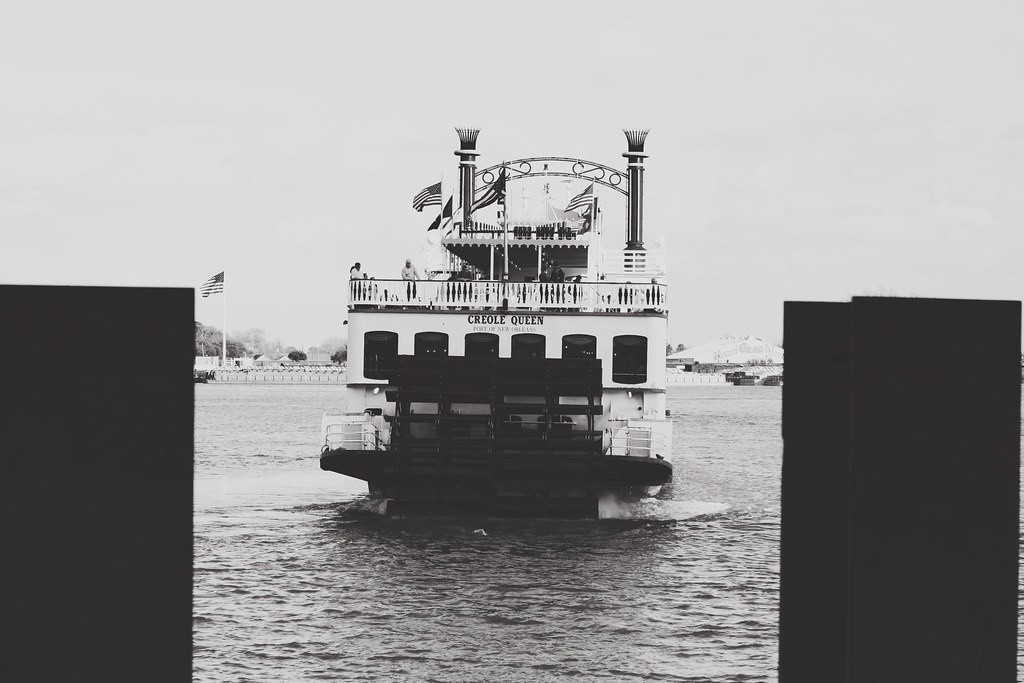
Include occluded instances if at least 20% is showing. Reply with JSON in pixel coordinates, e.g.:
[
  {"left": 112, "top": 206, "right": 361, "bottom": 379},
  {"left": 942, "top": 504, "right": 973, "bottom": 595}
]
[
  {"left": 552, "top": 262, "right": 566, "bottom": 312},
  {"left": 447, "top": 271, "right": 458, "bottom": 311},
  {"left": 539, "top": 267, "right": 551, "bottom": 312},
  {"left": 568, "top": 275, "right": 582, "bottom": 312},
  {"left": 458, "top": 265, "right": 472, "bottom": 311},
  {"left": 401, "top": 259, "right": 420, "bottom": 301},
  {"left": 350, "top": 262, "right": 378, "bottom": 301},
  {"left": 618, "top": 281, "right": 632, "bottom": 314},
  {"left": 647, "top": 278, "right": 659, "bottom": 312}
]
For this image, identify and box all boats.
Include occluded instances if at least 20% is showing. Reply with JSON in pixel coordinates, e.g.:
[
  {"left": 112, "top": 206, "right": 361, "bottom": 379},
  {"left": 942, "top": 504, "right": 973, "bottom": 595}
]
[{"left": 319, "top": 127, "right": 674, "bottom": 519}]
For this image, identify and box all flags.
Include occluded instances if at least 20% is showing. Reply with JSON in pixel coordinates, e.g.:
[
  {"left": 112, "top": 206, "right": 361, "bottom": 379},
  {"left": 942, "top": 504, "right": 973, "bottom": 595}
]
[
  {"left": 583, "top": 197, "right": 601, "bottom": 233},
  {"left": 564, "top": 183, "right": 593, "bottom": 213},
  {"left": 200, "top": 272, "right": 224, "bottom": 298},
  {"left": 413, "top": 182, "right": 442, "bottom": 212},
  {"left": 427, "top": 197, "right": 452, "bottom": 231},
  {"left": 470, "top": 169, "right": 506, "bottom": 214}
]
